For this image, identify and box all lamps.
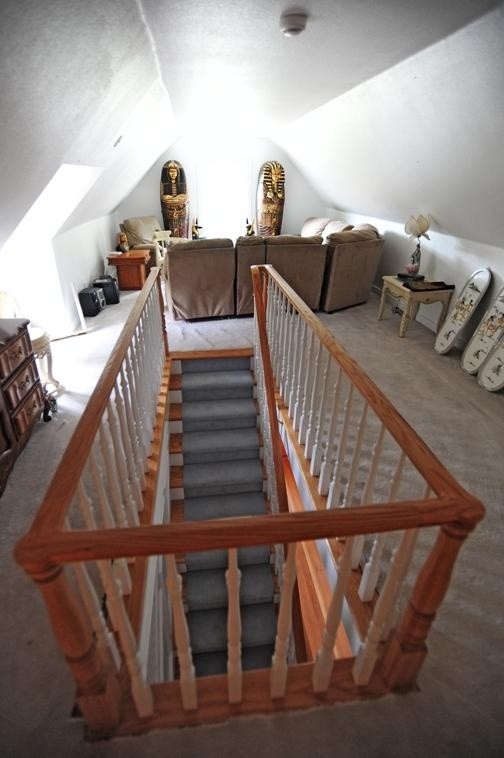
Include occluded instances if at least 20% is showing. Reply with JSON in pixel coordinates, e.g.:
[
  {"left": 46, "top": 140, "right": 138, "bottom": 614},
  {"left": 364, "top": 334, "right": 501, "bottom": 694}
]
[{"left": 121, "top": 213, "right": 386, "bottom": 323}]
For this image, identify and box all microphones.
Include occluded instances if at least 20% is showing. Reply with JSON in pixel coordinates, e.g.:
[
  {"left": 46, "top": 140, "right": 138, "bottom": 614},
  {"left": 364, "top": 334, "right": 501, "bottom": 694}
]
[{"left": 26, "top": 325, "right": 65, "bottom": 401}]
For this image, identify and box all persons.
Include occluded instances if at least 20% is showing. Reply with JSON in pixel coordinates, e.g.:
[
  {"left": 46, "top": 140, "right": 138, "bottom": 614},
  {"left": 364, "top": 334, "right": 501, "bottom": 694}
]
[{"left": 160, "top": 163, "right": 185, "bottom": 196}]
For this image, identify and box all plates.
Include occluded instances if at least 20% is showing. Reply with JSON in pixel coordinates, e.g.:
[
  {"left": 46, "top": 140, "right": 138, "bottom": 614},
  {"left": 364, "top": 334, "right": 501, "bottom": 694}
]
[{"left": 0, "top": 318, "right": 55, "bottom": 497}]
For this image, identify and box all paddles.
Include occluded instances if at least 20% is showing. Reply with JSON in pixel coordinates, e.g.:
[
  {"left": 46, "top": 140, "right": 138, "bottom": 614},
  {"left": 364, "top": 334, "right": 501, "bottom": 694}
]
[{"left": 178, "top": 355, "right": 282, "bottom": 678}]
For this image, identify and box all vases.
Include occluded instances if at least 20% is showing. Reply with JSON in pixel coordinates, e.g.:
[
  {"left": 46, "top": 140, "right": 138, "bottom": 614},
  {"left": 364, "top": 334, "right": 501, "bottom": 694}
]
[{"left": 403, "top": 213, "right": 431, "bottom": 244}]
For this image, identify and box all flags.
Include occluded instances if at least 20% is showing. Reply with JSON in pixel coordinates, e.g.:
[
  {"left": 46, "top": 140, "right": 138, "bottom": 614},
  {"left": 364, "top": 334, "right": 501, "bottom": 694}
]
[{"left": 404, "top": 244, "right": 421, "bottom": 276}]
